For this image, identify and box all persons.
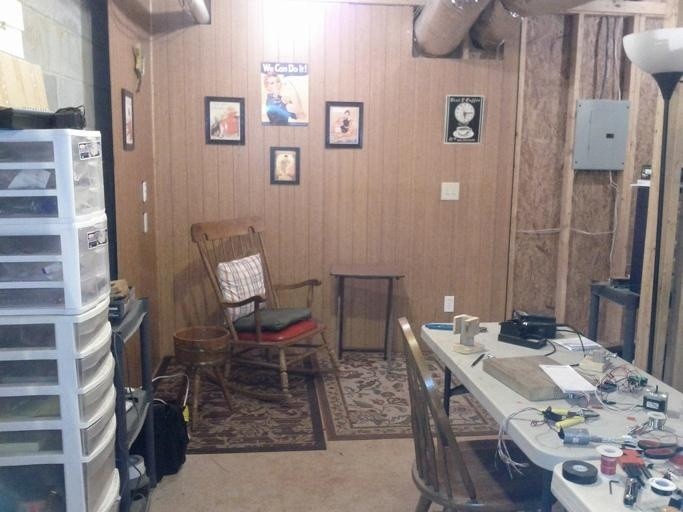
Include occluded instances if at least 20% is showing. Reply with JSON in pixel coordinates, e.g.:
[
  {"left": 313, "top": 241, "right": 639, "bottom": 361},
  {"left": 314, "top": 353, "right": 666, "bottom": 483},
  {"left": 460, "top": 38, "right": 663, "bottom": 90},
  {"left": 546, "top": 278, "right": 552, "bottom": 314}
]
[
  {"left": 264, "top": 74, "right": 304, "bottom": 123},
  {"left": 335, "top": 111, "right": 353, "bottom": 137}
]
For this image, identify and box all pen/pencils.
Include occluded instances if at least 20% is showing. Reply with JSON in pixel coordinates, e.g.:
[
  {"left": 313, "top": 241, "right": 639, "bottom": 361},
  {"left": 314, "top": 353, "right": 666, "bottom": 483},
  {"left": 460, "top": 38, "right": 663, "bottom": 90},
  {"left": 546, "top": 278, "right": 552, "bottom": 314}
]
[{"left": 471, "top": 354, "right": 485, "bottom": 366}]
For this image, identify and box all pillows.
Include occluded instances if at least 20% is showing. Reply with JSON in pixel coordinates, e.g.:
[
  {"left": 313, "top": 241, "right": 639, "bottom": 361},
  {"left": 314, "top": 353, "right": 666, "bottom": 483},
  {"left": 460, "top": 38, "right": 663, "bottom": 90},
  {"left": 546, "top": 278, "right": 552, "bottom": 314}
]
[
  {"left": 226, "top": 309, "right": 312, "bottom": 333},
  {"left": 215, "top": 253, "right": 268, "bottom": 329}
]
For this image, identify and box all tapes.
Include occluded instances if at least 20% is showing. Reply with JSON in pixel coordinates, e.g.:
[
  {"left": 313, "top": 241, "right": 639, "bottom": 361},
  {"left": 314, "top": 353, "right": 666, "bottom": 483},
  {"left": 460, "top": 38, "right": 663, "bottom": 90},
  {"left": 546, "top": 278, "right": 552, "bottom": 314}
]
[{"left": 562, "top": 460, "right": 599, "bottom": 486}]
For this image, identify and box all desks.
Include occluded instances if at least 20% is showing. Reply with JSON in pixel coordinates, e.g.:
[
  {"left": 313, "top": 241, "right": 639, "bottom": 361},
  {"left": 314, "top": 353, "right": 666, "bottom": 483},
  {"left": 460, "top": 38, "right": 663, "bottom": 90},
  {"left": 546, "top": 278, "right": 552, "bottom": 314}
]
[
  {"left": 548, "top": 459, "right": 683, "bottom": 512},
  {"left": 419, "top": 320, "right": 682, "bottom": 473}
]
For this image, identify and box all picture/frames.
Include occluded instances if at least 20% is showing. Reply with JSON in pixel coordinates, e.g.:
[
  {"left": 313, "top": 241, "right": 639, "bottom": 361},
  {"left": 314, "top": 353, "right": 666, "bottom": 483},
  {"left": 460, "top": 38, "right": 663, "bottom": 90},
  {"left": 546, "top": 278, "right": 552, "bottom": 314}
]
[
  {"left": 202, "top": 94, "right": 246, "bottom": 146},
  {"left": 267, "top": 145, "right": 302, "bottom": 187},
  {"left": 443, "top": 93, "right": 488, "bottom": 146},
  {"left": 323, "top": 100, "right": 365, "bottom": 151},
  {"left": 119, "top": 86, "right": 137, "bottom": 153}
]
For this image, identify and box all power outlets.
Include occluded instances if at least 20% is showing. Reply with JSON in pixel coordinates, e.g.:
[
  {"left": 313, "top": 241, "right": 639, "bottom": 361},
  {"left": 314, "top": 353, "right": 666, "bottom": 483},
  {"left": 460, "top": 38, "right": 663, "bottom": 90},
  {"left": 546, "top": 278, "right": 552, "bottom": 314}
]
[
  {"left": 442, "top": 294, "right": 456, "bottom": 313},
  {"left": 142, "top": 181, "right": 149, "bottom": 202},
  {"left": 439, "top": 181, "right": 461, "bottom": 201},
  {"left": 142, "top": 211, "right": 149, "bottom": 234}
]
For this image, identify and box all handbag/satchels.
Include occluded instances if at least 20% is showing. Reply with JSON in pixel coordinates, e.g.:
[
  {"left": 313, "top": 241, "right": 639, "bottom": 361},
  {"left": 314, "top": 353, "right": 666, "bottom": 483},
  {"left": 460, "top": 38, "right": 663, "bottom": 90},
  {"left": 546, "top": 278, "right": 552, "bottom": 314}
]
[{"left": 128, "top": 403, "right": 187, "bottom": 474}]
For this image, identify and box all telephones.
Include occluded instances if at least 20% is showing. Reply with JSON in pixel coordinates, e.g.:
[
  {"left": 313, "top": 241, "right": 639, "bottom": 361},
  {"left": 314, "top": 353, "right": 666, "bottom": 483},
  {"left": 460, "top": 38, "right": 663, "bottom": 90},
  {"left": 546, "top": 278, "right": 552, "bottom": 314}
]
[{"left": 498, "top": 309, "right": 558, "bottom": 349}]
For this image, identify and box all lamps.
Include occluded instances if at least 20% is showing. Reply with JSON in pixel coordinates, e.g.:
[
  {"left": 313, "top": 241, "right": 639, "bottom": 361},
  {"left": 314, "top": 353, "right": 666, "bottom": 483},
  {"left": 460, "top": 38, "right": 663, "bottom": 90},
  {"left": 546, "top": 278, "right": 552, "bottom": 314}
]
[{"left": 621, "top": 25, "right": 683, "bottom": 379}]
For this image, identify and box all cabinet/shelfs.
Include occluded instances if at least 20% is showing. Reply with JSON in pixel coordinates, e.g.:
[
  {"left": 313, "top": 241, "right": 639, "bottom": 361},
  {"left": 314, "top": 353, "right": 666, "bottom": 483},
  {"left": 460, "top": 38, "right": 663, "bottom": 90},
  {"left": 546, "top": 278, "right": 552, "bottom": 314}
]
[
  {"left": 0, "top": 128, "right": 106, "bottom": 225},
  {"left": 0, "top": 212, "right": 111, "bottom": 317},
  {"left": 0, "top": 294, "right": 123, "bottom": 512},
  {"left": 107, "top": 294, "right": 160, "bottom": 512}
]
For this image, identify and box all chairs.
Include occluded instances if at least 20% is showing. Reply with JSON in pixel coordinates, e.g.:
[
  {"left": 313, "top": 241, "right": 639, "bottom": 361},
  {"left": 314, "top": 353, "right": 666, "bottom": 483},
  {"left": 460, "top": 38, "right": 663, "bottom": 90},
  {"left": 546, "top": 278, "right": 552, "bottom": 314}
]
[
  {"left": 396, "top": 315, "right": 558, "bottom": 512},
  {"left": 190, "top": 215, "right": 342, "bottom": 393}
]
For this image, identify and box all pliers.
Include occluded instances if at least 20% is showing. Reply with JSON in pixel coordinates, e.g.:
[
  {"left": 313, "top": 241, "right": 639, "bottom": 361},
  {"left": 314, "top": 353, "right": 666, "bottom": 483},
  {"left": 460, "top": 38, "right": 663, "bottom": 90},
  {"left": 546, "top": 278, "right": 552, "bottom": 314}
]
[{"left": 552, "top": 408, "right": 599, "bottom": 428}]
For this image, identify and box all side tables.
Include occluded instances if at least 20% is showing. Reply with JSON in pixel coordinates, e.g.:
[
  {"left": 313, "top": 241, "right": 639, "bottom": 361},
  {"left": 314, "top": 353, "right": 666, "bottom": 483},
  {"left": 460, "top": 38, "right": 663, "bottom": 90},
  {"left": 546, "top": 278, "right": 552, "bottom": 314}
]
[{"left": 333, "top": 264, "right": 406, "bottom": 359}]
